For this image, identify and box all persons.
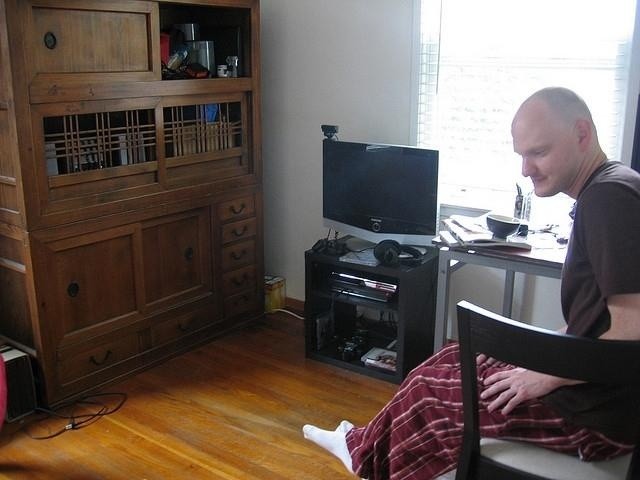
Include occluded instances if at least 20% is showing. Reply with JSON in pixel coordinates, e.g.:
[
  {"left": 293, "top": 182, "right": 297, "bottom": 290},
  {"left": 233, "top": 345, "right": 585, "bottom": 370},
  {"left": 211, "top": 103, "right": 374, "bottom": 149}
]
[{"left": 302, "top": 86, "right": 640, "bottom": 480}]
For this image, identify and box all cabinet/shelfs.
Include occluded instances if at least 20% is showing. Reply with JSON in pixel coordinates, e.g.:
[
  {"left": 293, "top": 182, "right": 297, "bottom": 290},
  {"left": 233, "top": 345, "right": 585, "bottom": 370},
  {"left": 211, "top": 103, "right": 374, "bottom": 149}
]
[
  {"left": 303, "top": 248, "right": 440, "bottom": 385},
  {"left": 0, "top": 1, "right": 264, "bottom": 409}
]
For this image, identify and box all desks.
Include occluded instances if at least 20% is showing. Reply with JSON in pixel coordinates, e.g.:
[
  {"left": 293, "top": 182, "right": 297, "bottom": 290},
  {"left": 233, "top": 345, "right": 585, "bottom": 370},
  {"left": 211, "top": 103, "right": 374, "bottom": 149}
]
[{"left": 432, "top": 232, "right": 573, "bottom": 354}]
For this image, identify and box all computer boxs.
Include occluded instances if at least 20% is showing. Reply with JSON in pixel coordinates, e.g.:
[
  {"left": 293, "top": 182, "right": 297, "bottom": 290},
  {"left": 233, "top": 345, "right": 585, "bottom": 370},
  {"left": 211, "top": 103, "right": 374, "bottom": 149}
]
[{"left": 0, "top": 344, "right": 37, "bottom": 423}]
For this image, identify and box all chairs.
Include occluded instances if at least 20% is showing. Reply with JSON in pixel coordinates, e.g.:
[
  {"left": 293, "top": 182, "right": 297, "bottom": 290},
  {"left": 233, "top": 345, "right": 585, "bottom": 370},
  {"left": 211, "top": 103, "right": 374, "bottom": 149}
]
[{"left": 453, "top": 297, "right": 637, "bottom": 480}]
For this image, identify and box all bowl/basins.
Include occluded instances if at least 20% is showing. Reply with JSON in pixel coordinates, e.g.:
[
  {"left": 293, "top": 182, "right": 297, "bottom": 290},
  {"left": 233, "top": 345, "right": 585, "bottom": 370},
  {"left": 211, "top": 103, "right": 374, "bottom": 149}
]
[{"left": 487, "top": 215, "right": 520, "bottom": 241}]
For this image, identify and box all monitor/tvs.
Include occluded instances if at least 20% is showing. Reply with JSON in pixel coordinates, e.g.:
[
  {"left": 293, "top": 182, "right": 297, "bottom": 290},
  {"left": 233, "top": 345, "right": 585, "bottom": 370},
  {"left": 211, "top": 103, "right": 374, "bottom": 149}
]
[{"left": 323, "top": 139, "right": 443, "bottom": 258}]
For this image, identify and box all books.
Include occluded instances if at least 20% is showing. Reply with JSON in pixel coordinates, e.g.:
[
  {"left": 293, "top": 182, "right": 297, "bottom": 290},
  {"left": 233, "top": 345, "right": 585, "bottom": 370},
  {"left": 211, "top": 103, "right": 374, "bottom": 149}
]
[{"left": 439, "top": 214, "right": 532, "bottom": 250}]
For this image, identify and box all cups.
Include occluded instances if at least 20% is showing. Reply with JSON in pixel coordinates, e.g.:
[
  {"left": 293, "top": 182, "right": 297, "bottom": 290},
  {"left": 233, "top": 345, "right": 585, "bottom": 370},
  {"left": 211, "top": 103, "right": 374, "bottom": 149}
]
[{"left": 176, "top": 22, "right": 215, "bottom": 79}]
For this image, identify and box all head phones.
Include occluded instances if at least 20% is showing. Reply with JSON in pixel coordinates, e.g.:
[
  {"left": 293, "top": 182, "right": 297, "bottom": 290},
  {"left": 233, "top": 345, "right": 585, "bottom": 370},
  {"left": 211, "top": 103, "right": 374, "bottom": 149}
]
[{"left": 373, "top": 240, "right": 424, "bottom": 267}]
